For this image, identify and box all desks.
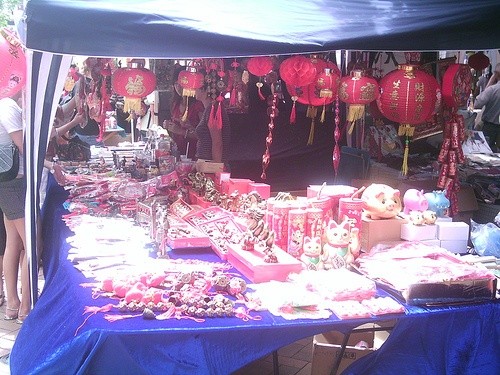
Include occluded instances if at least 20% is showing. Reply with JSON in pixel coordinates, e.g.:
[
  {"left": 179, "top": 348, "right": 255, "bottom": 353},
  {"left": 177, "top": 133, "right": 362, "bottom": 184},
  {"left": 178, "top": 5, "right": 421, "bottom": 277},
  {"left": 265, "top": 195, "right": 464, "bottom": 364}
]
[{"left": 10, "top": 170, "right": 500, "bottom": 375}]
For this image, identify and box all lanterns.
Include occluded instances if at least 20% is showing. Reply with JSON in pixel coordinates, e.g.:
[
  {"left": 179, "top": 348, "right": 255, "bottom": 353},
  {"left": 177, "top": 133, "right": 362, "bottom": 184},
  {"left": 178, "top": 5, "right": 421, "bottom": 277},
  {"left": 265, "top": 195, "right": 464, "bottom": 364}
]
[
  {"left": 280, "top": 53, "right": 380, "bottom": 146},
  {"left": 468, "top": 53, "right": 490, "bottom": 72},
  {"left": 84, "top": 58, "right": 157, "bottom": 114},
  {"left": 375, "top": 64, "right": 441, "bottom": 176},
  {"left": 0, "top": 27, "right": 27, "bottom": 100},
  {"left": 247, "top": 56, "right": 274, "bottom": 100},
  {"left": 177, "top": 61, "right": 204, "bottom": 97}
]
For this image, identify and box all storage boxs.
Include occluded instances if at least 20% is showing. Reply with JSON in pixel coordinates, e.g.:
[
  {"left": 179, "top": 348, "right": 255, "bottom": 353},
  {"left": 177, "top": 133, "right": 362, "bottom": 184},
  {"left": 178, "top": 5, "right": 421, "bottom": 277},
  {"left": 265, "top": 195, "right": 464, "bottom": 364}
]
[
  {"left": 312, "top": 323, "right": 390, "bottom": 375},
  {"left": 359, "top": 211, "right": 469, "bottom": 253},
  {"left": 188, "top": 159, "right": 270, "bottom": 210}
]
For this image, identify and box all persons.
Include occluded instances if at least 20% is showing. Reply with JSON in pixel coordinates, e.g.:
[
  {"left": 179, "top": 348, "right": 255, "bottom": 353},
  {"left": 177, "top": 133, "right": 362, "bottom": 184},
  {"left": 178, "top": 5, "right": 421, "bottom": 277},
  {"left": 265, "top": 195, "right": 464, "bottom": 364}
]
[
  {"left": 469, "top": 63, "right": 500, "bottom": 153},
  {"left": 163, "top": 66, "right": 205, "bottom": 161},
  {"left": 59, "top": 64, "right": 156, "bottom": 146},
  {"left": 194, "top": 68, "right": 231, "bottom": 177},
  {"left": 0, "top": 88, "right": 83, "bottom": 323}
]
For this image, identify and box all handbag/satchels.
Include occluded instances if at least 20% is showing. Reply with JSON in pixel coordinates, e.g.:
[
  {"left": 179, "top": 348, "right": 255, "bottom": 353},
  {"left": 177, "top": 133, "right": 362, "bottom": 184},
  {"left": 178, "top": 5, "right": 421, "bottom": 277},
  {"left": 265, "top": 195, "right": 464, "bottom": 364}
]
[{"left": 59, "top": 135, "right": 92, "bottom": 161}]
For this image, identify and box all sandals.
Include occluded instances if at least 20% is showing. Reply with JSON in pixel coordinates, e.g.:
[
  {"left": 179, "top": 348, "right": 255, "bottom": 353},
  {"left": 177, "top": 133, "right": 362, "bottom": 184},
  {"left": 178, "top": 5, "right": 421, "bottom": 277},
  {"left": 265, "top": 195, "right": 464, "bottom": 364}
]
[{"left": 4, "top": 307, "right": 29, "bottom": 325}]
